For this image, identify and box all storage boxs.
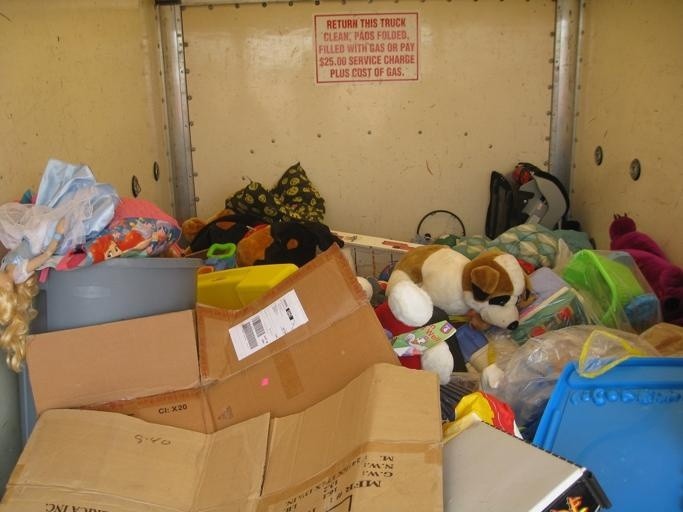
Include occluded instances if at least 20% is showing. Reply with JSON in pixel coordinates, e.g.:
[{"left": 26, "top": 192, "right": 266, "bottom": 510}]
[{"left": 38, "top": 258, "right": 204, "bottom": 332}]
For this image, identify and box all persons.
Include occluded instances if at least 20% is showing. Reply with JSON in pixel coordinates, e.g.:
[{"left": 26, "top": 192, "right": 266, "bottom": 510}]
[{"left": 0, "top": 159, "right": 113, "bottom": 376}]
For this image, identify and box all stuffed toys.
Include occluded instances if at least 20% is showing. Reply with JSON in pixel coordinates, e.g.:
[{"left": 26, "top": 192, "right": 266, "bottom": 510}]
[
  {"left": 183, "top": 210, "right": 314, "bottom": 264},
  {"left": 357, "top": 213, "right": 683, "bottom": 384}
]
